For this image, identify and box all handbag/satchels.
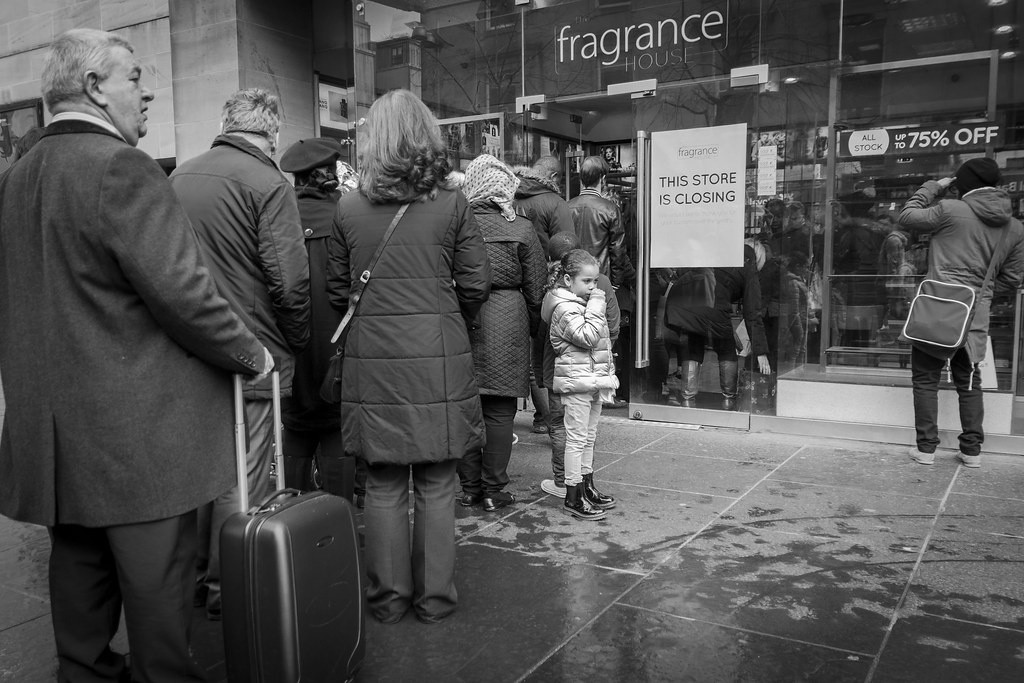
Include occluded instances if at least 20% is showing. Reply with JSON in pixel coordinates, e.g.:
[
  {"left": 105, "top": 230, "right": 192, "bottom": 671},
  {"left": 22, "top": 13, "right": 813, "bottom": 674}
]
[
  {"left": 904, "top": 279, "right": 976, "bottom": 347},
  {"left": 654, "top": 281, "right": 680, "bottom": 341}
]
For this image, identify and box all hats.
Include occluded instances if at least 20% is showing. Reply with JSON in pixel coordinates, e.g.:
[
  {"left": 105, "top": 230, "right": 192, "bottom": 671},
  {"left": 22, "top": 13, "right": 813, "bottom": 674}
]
[
  {"left": 279, "top": 137, "right": 343, "bottom": 174},
  {"left": 955, "top": 158, "right": 1001, "bottom": 194}
]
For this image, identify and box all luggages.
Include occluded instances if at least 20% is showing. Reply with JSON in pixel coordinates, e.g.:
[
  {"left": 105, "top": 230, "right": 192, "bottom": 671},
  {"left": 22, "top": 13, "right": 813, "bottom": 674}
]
[{"left": 216, "top": 356, "right": 366, "bottom": 683}]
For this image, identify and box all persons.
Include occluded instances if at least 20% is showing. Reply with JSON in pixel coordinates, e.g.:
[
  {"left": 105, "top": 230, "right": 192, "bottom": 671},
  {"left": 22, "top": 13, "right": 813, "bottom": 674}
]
[
  {"left": 280, "top": 138, "right": 369, "bottom": 508},
  {"left": 812, "top": 128, "right": 828, "bottom": 158},
  {"left": 540, "top": 250, "right": 620, "bottom": 521},
  {"left": 325, "top": 88, "right": 490, "bottom": 624},
  {"left": 751, "top": 132, "right": 784, "bottom": 162},
  {"left": 569, "top": 156, "right": 636, "bottom": 408},
  {"left": 551, "top": 141, "right": 559, "bottom": 157},
  {"left": 663, "top": 239, "right": 770, "bottom": 410},
  {"left": 606, "top": 148, "right": 615, "bottom": 162},
  {"left": 512, "top": 155, "right": 574, "bottom": 433},
  {"left": 0, "top": 29, "right": 275, "bottom": 683},
  {"left": 168, "top": 87, "right": 311, "bottom": 620},
  {"left": 898, "top": 158, "right": 1024, "bottom": 468},
  {"left": 644, "top": 268, "right": 678, "bottom": 403},
  {"left": 530, "top": 231, "right": 619, "bottom": 498},
  {"left": 755, "top": 191, "right": 929, "bottom": 411},
  {"left": 453, "top": 155, "right": 549, "bottom": 511}
]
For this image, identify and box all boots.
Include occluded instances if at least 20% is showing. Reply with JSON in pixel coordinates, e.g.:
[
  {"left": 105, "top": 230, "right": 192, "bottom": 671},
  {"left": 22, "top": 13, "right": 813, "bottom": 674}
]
[
  {"left": 680, "top": 360, "right": 702, "bottom": 407},
  {"left": 564, "top": 480, "right": 608, "bottom": 521},
  {"left": 581, "top": 471, "right": 616, "bottom": 510},
  {"left": 719, "top": 360, "right": 738, "bottom": 411}
]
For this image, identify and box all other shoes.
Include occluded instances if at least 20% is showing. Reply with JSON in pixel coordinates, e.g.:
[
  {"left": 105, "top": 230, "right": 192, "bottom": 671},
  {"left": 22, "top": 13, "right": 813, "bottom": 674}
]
[
  {"left": 540, "top": 479, "right": 567, "bottom": 499},
  {"left": 461, "top": 493, "right": 477, "bottom": 506},
  {"left": 483, "top": 493, "right": 514, "bottom": 510}
]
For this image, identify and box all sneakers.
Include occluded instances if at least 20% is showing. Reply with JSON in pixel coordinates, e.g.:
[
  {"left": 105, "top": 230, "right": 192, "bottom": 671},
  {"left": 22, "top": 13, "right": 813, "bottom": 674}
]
[
  {"left": 909, "top": 448, "right": 935, "bottom": 464},
  {"left": 954, "top": 451, "right": 981, "bottom": 468}
]
